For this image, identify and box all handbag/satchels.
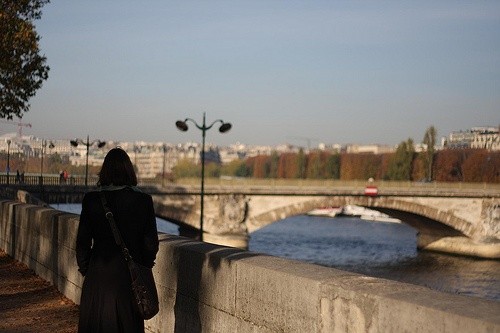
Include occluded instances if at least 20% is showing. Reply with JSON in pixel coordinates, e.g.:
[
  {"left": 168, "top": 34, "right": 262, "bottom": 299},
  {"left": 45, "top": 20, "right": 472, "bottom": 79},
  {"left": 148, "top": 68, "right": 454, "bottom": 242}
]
[{"left": 126, "top": 254, "right": 159, "bottom": 319}]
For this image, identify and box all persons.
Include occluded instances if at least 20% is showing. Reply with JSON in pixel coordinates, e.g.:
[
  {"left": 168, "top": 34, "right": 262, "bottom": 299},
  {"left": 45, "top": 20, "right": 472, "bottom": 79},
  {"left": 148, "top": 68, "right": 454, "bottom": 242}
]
[
  {"left": 20, "top": 170, "right": 26, "bottom": 183},
  {"left": 15, "top": 168, "right": 20, "bottom": 183},
  {"left": 74, "top": 147, "right": 159, "bottom": 333},
  {"left": 59, "top": 168, "right": 68, "bottom": 184}
]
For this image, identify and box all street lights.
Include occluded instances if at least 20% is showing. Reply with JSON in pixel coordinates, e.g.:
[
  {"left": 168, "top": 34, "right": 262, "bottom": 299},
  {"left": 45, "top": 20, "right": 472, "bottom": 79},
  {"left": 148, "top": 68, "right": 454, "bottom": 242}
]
[
  {"left": 70, "top": 135, "right": 106, "bottom": 196},
  {"left": 175, "top": 113, "right": 232, "bottom": 240},
  {"left": 5, "top": 139, "right": 11, "bottom": 199},
  {"left": 38, "top": 138, "right": 55, "bottom": 201}
]
